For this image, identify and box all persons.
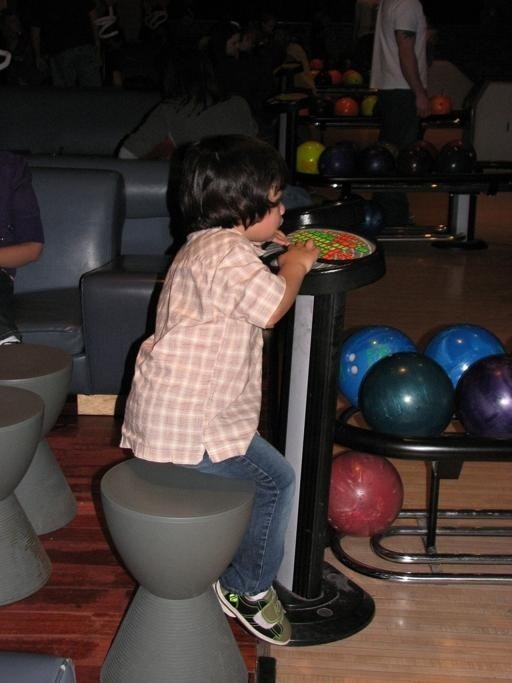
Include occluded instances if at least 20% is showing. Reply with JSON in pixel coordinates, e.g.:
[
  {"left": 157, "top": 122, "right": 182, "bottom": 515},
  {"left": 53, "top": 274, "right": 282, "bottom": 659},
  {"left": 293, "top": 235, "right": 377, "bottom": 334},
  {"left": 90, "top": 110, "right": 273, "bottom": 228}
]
[
  {"left": 0, "top": 151, "right": 43, "bottom": 344},
  {"left": 118, "top": 136, "right": 322, "bottom": 648},
  {"left": 369, "top": 0, "right": 432, "bottom": 149}
]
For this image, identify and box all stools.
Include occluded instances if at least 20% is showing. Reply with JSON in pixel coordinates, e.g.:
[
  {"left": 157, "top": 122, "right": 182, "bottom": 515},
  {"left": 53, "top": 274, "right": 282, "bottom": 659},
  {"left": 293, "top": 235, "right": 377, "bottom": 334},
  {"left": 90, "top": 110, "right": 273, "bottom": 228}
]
[
  {"left": 0, "top": 384, "right": 55, "bottom": 608},
  {"left": 2, "top": 342, "right": 77, "bottom": 532},
  {"left": 92, "top": 457, "right": 257, "bottom": 683}
]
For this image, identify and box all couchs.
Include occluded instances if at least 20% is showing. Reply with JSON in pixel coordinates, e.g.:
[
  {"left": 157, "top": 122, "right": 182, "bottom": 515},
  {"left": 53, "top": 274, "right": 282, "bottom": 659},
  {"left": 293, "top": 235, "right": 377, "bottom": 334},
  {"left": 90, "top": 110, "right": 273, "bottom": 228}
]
[{"left": 0, "top": 77, "right": 266, "bottom": 390}]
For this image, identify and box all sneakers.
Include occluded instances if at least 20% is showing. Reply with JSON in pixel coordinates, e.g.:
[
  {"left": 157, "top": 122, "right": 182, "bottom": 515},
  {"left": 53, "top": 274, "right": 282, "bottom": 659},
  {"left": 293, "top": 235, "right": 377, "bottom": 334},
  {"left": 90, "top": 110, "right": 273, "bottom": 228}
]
[{"left": 216, "top": 580, "right": 292, "bottom": 646}]
